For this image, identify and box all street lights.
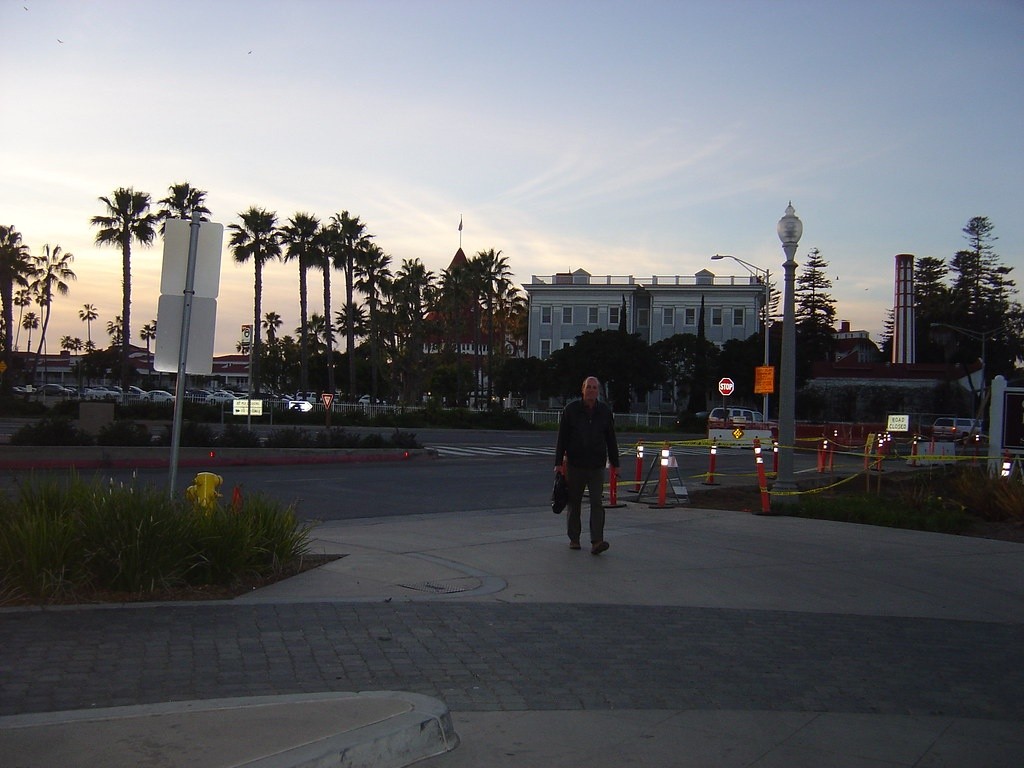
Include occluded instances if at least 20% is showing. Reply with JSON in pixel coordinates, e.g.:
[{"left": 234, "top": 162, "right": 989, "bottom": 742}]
[
  {"left": 929, "top": 318, "right": 1022, "bottom": 426},
  {"left": 711, "top": 254, "right": 771, "bottom": 423},
  {"left": 770, "top": 199, "right": 804, "bottom": 505},
  {"left": 243, "top": 320, "right": 253, "bottom": 436}
]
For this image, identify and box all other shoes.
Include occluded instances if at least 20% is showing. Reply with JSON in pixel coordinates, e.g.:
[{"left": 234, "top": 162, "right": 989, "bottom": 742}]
[
  {"left": 570, "top": 538, "right": 581, "bottom": 548},
  {"left": 591, "top": 540, "right": 609, "bottom": 554}
]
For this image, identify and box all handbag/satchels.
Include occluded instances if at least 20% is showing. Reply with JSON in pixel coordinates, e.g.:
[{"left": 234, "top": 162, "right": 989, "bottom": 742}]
[{"left": 551, "top": 471, "right": 567, "bottom": 514}]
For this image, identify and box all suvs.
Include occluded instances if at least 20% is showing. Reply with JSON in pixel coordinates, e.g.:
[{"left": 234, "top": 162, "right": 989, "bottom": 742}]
[
  {"left": 708, "top": 407, "right": 763, "bottom": 423},
  {"left": 932, "top": 418, "right": 983, "bottom": 440}
]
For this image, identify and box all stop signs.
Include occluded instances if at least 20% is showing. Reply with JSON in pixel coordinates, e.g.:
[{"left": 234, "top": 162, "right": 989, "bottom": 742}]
[{"left": 718, "top": 377, "right": 734, "bottom": 396}]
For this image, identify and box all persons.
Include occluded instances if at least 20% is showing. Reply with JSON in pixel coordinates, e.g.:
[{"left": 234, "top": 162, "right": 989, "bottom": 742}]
[{"left": 552, "top": 377, "right": 621, "bottom": 554}]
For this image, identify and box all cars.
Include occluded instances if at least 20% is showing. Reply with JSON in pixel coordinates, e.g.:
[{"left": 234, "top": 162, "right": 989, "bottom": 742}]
[{"left": 12, "top": 380, "right": 381, "bottom": 408}]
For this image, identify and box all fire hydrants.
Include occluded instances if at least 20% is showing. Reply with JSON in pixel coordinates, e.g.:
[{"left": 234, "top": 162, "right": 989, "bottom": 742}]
[{"left": 184, "top": 472, "right": 225, "bottom": 530}]
